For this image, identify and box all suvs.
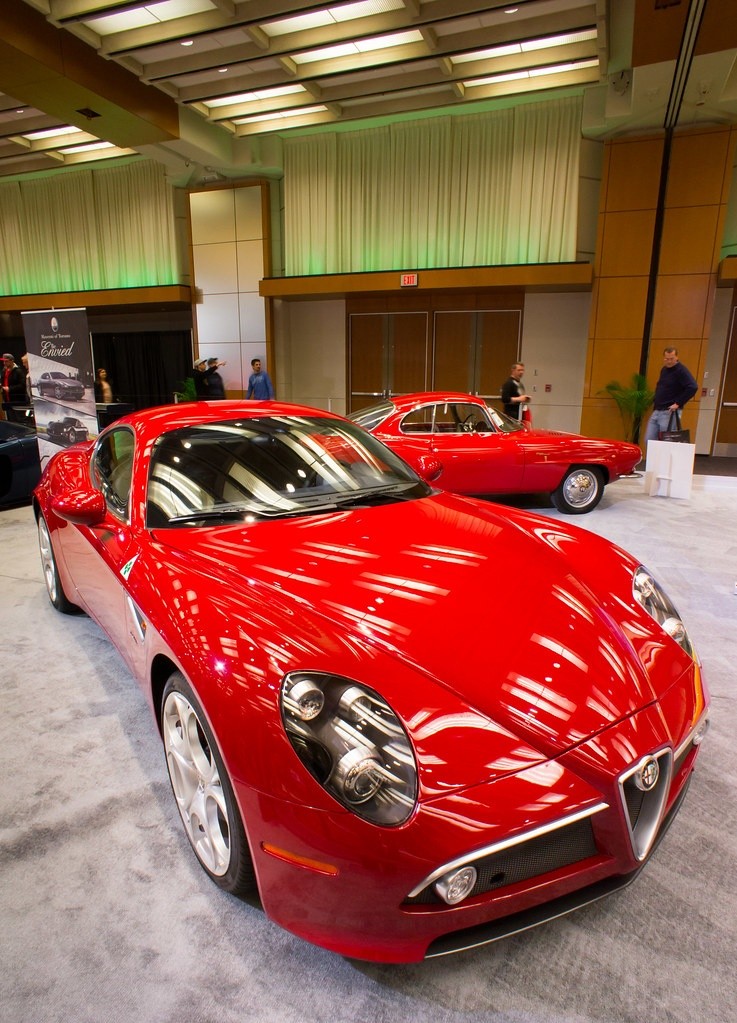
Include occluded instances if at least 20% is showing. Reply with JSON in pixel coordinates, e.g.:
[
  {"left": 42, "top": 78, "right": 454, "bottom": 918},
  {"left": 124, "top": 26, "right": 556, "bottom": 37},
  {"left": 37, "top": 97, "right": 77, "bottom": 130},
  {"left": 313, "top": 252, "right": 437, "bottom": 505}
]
[{"left": 46, "top": 417, "right": 90, "bottom": 444}]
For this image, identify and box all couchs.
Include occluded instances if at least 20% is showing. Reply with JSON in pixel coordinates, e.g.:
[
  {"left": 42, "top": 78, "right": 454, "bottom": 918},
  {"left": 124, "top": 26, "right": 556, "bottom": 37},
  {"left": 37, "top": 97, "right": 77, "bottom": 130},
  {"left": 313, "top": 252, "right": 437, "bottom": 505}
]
[{"left": 0, "top": 418, "right": 44, "bottom": 512}]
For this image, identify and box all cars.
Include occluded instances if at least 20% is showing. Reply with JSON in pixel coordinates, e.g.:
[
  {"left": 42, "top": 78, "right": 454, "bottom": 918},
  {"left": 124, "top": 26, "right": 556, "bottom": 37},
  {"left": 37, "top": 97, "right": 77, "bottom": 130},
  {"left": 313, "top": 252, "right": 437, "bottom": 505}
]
[{"left": 34, "top": 372, "right": 85, "bottom": 400}]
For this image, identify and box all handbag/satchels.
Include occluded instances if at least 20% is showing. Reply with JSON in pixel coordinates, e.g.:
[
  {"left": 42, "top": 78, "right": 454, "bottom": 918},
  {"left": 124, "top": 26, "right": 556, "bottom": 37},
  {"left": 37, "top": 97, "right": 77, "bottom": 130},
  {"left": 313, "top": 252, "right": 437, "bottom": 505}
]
[
  {"left": 522, "top": 400, "right": 531, "bottom": 423},
  {"left": 658, "top": 407, "right": 691, "bottom": 443}
]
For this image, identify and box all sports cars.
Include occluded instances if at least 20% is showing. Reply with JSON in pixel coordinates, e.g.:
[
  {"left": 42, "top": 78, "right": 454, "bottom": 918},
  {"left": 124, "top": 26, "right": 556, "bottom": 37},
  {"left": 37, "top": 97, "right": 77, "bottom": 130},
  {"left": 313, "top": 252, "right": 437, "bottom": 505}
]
[
  {"left": 294, "top": 390, "right": 643, "bottom": 516},
  {"left": 28, "top": 398, "right": 711, "bottom": 966}
]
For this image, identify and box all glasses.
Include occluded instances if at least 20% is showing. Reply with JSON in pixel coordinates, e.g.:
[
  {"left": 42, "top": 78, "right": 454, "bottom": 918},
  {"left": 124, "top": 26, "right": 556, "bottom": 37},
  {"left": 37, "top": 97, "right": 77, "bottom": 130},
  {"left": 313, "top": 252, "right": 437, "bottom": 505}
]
[{"left": 664, "top": 357, "right": 675, "bottom": 361}]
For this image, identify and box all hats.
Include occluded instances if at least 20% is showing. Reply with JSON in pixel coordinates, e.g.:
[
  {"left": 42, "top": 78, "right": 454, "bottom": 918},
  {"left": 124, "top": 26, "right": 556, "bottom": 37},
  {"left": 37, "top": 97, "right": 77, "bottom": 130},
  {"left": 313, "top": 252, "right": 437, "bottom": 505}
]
[
  {"left": 194, "top": 359, "right": 207, "bottom": 367},
  {"left": 208, "top": 357, "right": 218, "bottom": 365},
  {"left": 0, "top": 353, "right": 14, "bottom": 361}
]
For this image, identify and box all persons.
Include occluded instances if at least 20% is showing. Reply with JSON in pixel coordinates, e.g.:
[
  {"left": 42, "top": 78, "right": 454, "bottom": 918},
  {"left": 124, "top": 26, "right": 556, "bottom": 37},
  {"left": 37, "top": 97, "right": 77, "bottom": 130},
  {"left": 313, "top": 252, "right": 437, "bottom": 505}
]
[
  {"left": 94, "top": 369, "right": 115, "bottom": 428},
  {"left": 0, "top": 354, "right": 25, "bottom": 422},
  {"left": 207, "top": 357, "right": 225, "bottom": 400},
  {"left": 245, "top": 358, "right": 275, "bottom": 400},
  {"left": 644, "top": 347, "right": 698, "bottom": 449},
  {"left": 192, "top": 359, "right": 227, "bottom": 401},
  {"left": 22, "top": 354, "right": 34, "bottom": 405},
  {"left": 500, "top": 362, "right": 531, "bottom": 424}
]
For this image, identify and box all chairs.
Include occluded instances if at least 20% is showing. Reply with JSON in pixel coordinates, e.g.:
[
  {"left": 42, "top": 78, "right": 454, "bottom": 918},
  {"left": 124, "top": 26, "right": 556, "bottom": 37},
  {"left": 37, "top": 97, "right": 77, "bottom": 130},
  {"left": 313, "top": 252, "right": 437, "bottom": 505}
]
[
  {"left": 212, "top": 434, "right": 311, "bottom": 504},
  {"left": 152, "top": 436, "right": 193, "bottom": 474}
]
